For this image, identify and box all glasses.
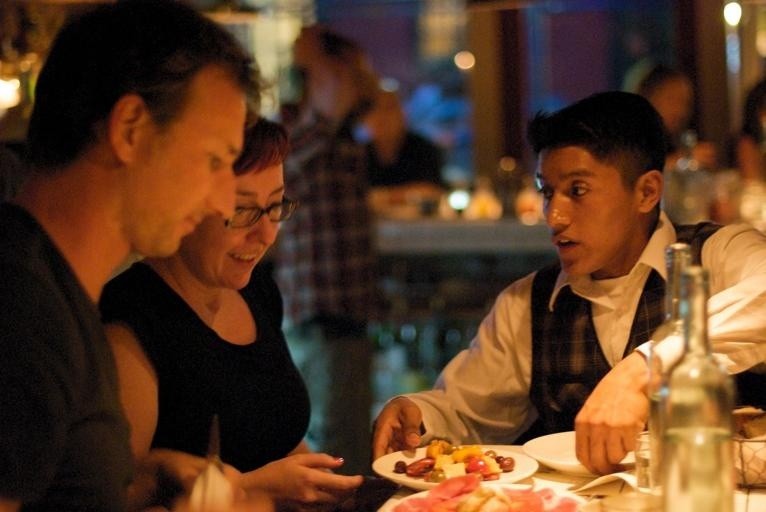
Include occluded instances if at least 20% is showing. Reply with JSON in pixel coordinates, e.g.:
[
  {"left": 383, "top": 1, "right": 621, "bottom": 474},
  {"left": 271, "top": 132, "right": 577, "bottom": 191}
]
[{"left": 223, "top": 195, "right": 298, "bottom": 226}]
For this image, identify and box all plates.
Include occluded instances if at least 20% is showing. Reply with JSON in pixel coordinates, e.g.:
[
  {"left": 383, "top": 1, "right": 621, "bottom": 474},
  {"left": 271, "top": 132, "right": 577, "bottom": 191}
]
[
  {"left": 384, "top": 484, "right": 589, "bottom": 512},
  {"left": 520, "top": 430, "right": 636, "bottom": 475},
  {"left": 371, "top": 441, "right": 539, "bottom": 493}
]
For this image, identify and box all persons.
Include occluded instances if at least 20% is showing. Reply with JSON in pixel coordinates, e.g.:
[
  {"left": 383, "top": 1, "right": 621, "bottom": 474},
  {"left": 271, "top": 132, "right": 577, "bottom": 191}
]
[
  {"left": 737, "top": 76, "right": 765, "bottom": 177},
  {"left": 363, "top": 91, "right": 447, "bottom": 183},
  {"left": 626, "top": 61, "right": 699, "bottom": 154},
  {"left": 367, "top": 90, "right": 766, "bottom": 476},
  {"left": 271, "top": 26, "right": 379, "bottom": 464},
  {"left": 0, "top": 0, "right": 279, "bottom": 511},
  {"left": 99, "top": 110, "right": 365, "bottom": 511}
]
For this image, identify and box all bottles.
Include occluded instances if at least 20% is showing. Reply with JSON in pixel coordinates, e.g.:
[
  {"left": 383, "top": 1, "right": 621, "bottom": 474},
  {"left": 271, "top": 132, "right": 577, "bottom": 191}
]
[{"left": 650, "top": 243, "right": 736, "bottom": 512}]
